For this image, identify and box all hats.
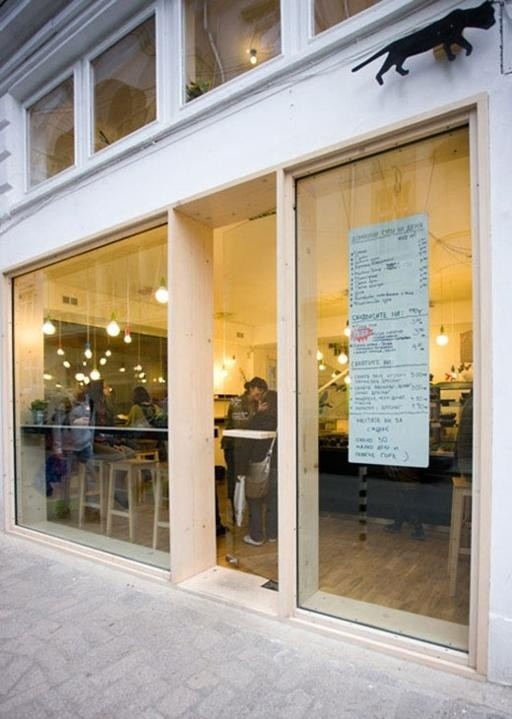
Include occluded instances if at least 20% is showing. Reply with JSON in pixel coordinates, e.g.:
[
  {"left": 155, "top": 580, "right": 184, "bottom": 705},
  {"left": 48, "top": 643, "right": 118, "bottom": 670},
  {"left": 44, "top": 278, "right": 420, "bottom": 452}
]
[{"left": 244, "top": 377, "right": 267, "bottom": 391}]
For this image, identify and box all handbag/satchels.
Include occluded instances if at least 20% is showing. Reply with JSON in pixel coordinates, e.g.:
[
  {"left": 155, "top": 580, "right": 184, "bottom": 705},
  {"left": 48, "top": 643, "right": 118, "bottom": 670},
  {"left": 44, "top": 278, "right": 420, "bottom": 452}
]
[{"left": 247, "top": 456, "right": 269, "bottom": 499}]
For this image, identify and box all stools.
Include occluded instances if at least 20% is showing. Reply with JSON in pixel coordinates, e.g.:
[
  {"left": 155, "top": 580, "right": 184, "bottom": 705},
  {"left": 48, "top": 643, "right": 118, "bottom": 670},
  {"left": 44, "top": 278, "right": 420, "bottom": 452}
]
[
  {"left": 445, "top": 476, "right": 472, "bottom": 597},
  {"left": 55, "top": 437, "right": 171, "bottom": 551}
]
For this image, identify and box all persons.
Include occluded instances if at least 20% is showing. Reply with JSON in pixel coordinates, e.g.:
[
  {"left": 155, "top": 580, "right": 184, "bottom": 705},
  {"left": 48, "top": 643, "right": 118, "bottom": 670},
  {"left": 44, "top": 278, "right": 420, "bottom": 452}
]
[
  {"left": 51, "top": 375, "right": 100, "bottom": 520},
  {"left": 159, "top": 393, "right": 168, "bottom": 409},
  {"left": 383, "top": 466, "right": 425, "bottom": 541},
  {"left": 237, "top": 391, "right": 277, "bottom": 546},
  {"left": 220, "top": 377, "right": 269, "bottom": 525},
  {"left": 455, "top": 382, "right": 473, "bottom": 525},
  {"left": 120, "top": 386, "right": 168, "bottom": 482},
  {"left": 87, "top": 378, "right": 137, "bottom": 510}
]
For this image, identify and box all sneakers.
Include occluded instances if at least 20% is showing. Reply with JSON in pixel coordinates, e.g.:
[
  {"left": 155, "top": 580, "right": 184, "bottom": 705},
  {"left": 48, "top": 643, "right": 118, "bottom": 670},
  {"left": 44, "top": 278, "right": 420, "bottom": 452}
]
[{"left": 243, "top": 536, "right": 276, "bottom": 546}]
